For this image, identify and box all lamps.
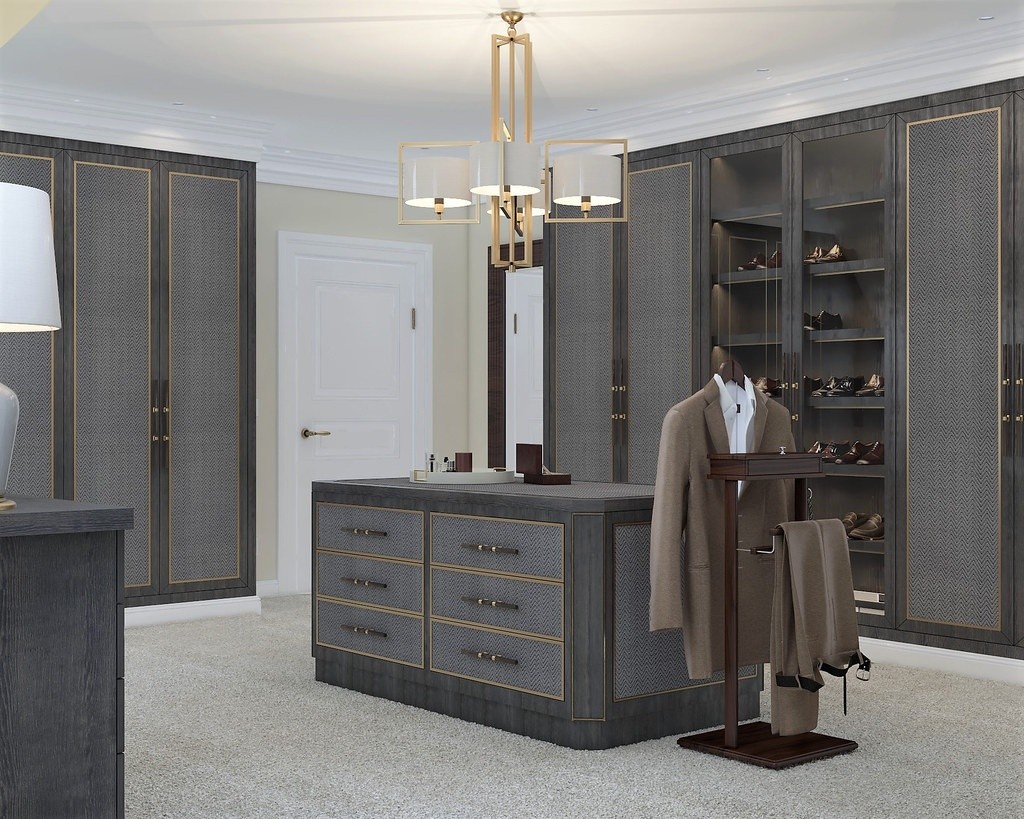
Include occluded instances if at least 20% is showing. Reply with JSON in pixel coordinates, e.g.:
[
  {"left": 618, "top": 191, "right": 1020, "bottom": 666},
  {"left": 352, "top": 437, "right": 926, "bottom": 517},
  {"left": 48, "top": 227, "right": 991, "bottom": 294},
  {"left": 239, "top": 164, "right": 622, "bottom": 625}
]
[
  {"left": 0, "top": 181, "right": 63, "bottom": 510},
  {"left": 399, "top": 10, "right": 628, "bottom": 271}
]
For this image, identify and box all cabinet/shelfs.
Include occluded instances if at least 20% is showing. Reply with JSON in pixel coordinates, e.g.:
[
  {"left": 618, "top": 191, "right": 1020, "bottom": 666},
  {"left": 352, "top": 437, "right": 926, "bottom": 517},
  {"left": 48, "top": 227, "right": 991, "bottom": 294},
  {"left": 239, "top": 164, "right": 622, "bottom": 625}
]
[
  {"left": 698, "top": 115, "right": 894, "bottom": 630},
  {"left": 311, "top": 476, "right": 763, "bottom": 750},
  {"left": 63, "top": 150, "right": 247, "bottom": 597},
  {"left": 894, "top": 90, "right": 1024, "bottom": 648},
  {"left": 0, "top": 143, "right": 65, "bottom": 500},
  {"left": 0, "top": 494, "right": 135, "bottom": 819},
  {"left": 549, "top": 152, "right": 697, "bottom": 488}
]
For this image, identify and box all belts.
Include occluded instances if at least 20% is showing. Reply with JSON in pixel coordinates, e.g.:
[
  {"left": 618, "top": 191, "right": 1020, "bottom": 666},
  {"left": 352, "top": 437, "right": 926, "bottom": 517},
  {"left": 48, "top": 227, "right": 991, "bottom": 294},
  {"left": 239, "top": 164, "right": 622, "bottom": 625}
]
[{"left": 775, "top": 651, "right": 873, "bottom": 715}]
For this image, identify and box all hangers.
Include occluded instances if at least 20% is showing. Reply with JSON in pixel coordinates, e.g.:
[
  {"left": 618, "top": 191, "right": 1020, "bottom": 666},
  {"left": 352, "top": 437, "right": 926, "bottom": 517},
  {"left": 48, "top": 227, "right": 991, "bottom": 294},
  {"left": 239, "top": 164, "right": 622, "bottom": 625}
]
[{"left": 716, "top": 359, "right": 746, "bottom": 414}]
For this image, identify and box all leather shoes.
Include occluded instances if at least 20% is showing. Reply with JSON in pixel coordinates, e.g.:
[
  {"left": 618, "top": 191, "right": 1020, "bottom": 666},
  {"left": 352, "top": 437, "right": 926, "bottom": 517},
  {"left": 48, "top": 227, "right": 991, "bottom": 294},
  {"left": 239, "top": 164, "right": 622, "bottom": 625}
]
[
  {"left": 737, "top": 250, "right": 784, "bottom": 270},
  {"left": 804, "top": 310, "right": 842, "bottom": 330},
  {"left": 805, "top": 438, "right": 885, "bottom": 466},
  {"left": 840, "top": 511, "right": 885, "bottom": 541},
  {"left": 747, "top": 374, "right": 888, "bottom": 396},
  {"left": 804, "top": 246, "right": 846, "bottom": 262}
]
